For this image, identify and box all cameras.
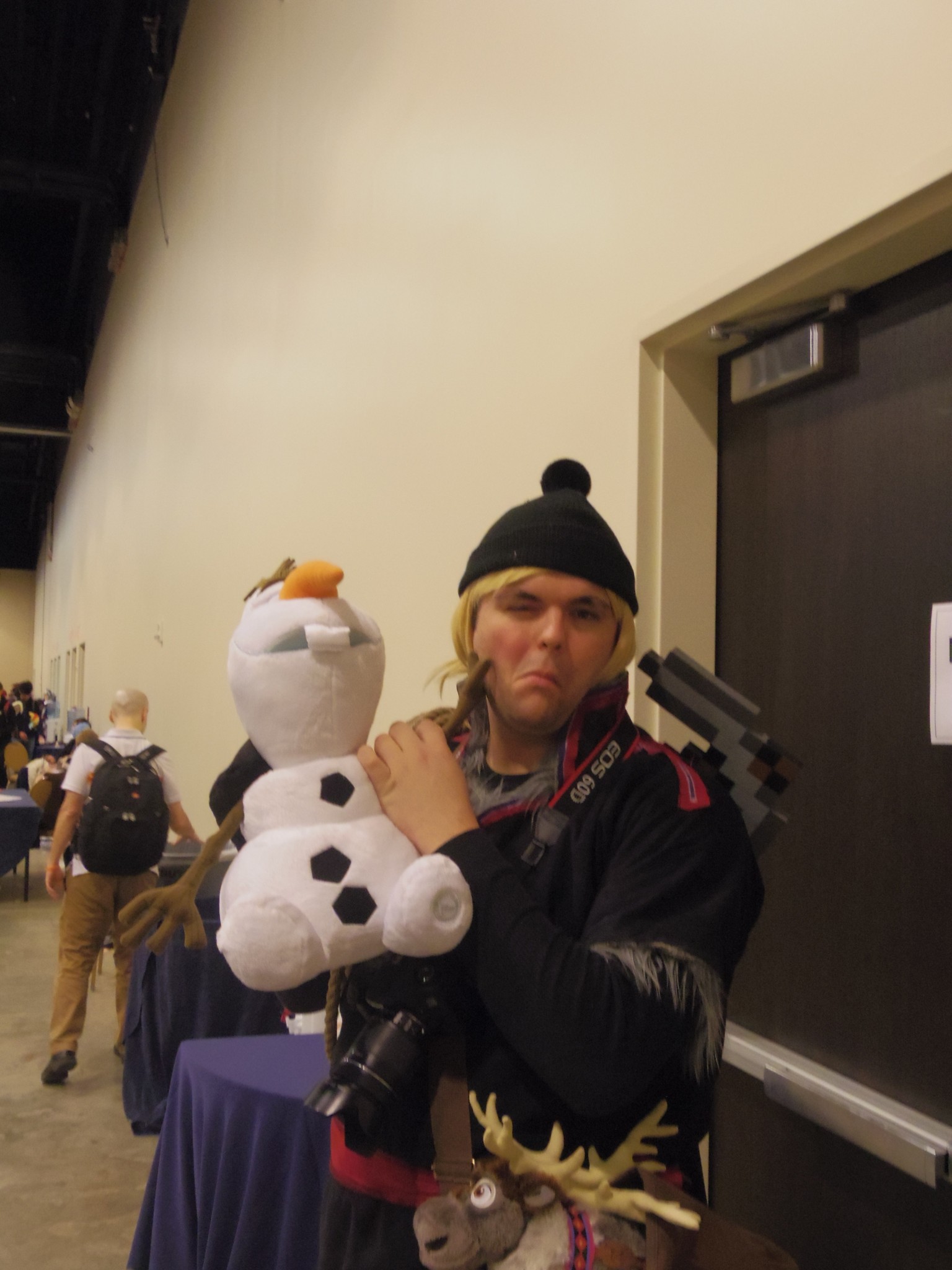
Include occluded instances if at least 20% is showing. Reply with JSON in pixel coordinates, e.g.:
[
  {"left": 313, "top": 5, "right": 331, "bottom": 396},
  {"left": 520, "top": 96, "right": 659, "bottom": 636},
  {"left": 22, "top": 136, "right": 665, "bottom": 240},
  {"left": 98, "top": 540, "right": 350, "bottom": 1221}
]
[{"left": 306, "top": 956, "right": 492, "bottom": 1158}]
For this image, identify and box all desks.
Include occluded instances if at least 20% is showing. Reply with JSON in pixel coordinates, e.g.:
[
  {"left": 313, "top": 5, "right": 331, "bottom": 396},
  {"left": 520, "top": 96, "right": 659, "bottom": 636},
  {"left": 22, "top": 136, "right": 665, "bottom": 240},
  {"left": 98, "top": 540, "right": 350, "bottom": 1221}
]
[
  {"left": 127, "top": 1034, "right": 331, "bottom": 1270},
  {"left": 0, "top": 788, "right": 43, "bottom": 902},
  {"left": 122, "top": 898, "right": 330, "bottom": 1136}
]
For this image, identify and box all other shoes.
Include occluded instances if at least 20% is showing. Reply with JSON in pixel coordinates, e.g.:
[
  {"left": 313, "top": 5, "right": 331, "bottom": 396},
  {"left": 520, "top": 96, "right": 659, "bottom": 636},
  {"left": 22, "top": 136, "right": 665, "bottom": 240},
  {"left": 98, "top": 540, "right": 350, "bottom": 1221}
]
[{"left": 40, "top": 1051, "right": 78, "bottom": 1087}]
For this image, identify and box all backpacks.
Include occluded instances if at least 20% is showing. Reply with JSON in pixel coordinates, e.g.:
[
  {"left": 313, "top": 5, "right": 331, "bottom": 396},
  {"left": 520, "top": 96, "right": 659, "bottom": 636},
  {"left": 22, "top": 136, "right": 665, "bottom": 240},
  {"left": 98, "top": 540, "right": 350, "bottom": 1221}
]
[{"left": 80, "top": 737, "right": 170, "bottom": 877}]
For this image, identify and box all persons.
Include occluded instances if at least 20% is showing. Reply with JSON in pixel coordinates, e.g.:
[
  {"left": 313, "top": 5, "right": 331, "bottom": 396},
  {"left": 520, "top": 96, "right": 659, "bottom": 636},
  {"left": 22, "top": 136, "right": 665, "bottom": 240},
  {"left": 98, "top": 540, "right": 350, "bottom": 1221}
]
[
  {"left": 41, "top": 687, "right": 198, "bottom": 1084},
  {"left": 208, "top": 453, "right": 761, "bottom": 1270},
  {"left": 0, "top": 679, "right": 92, "bottom": 787}
]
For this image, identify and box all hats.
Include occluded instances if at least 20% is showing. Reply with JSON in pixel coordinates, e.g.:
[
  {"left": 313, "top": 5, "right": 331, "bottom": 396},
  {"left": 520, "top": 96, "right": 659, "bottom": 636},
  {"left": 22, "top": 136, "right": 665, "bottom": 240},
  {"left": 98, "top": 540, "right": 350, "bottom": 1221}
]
[{"left": 458, "top": 457, "right": 639, "bottom": 617}]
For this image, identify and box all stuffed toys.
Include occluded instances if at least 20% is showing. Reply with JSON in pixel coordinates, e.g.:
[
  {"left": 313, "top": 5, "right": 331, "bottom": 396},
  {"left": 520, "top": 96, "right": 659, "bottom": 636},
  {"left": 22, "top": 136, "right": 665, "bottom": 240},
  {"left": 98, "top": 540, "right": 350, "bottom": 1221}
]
[
  {"left": 421, "top": 1092, "right": 703, "bottom": 1270},
  {"left": 117, "top": 559, "right": 490, "bottom": 995}
]
